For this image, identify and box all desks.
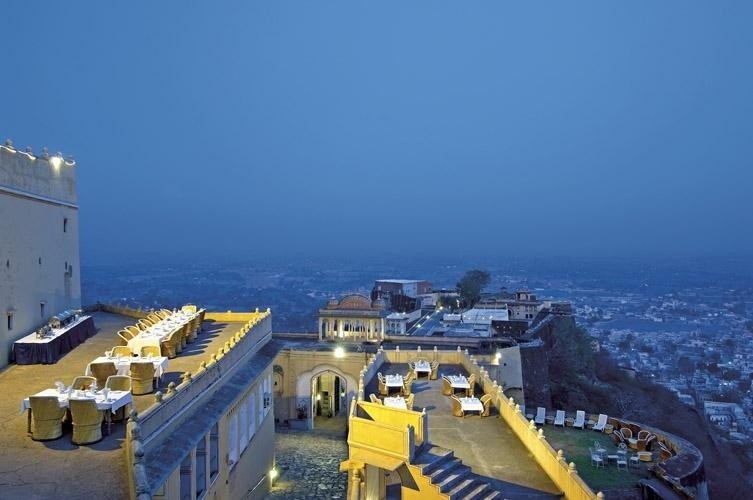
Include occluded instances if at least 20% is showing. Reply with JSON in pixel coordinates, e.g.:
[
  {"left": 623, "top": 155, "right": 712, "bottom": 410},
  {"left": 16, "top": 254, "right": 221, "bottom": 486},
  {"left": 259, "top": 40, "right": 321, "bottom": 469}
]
[
  {"left": 23, "top": 388, "right": 131, "bottom": 435},
  {"left": 86, "top": 356, "right": 168, "bottom": 389},
  {"left": 12, "top": 316, "right": 93, "bottom": 365}
]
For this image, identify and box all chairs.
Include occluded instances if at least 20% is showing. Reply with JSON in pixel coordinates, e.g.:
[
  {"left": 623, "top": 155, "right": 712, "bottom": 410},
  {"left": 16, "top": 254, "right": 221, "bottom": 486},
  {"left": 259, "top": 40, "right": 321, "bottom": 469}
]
[
  {"left": 518, "top": 404, "right": 608, "bottom": 432},
  {"left": 588, "top": 428, "right": 672, "bottom": 473},
  {"left": 29, "top": 346, "right": 162, "bottom": 444},
  {"left": 369, "top": 371, "right": 415, "bottom": 411},
  {"left": 116, "top": 305, "right": 206, "bottom": 359},
  {"left": 407, "top": 357, "right": 439, "bottom": 380},
  {"left": 441, "top": 374, "right": 494, "bottom": 416}
]
